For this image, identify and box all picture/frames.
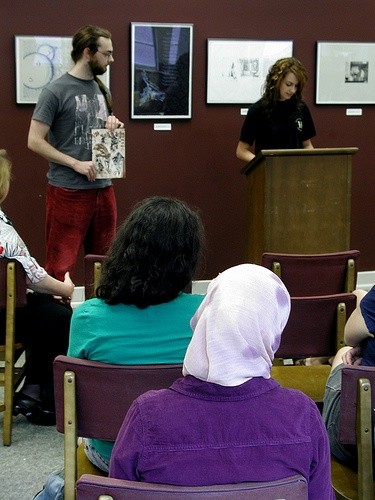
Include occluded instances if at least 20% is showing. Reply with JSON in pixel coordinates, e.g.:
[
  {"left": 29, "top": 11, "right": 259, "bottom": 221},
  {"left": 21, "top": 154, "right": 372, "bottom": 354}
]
[
  {"left": 315, "top": 40, "right": 375, "bottom": 107},
  {"left": 15, "top": 35, "right": 111, "bottom": 104},
  {"left": 130, "top": 22, "right": 194, "bottom": 119},
  {"left": 206, "top": 37, "right": 294, "bottom": 104}
]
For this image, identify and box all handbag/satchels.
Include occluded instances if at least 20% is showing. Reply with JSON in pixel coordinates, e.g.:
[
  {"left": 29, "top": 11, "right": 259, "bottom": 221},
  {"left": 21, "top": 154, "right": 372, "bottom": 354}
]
[{"left": 32, "top": 468, "right": 65, "bottom": 500}]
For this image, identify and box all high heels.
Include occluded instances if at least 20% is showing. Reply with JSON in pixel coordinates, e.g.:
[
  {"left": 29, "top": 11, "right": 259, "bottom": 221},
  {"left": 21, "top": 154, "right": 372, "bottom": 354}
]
[{"left": 11, "top": 393, "right": 57, "bottom": 425}]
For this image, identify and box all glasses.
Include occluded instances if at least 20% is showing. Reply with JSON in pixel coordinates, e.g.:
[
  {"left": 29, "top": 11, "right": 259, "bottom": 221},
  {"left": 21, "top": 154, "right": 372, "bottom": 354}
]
[{"left": 96, "top": 49, "right": 114, "bottom": 60}]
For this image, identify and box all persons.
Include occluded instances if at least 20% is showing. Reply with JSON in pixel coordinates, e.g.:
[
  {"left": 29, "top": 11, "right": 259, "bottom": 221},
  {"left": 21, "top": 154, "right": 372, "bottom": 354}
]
[
  {"left": 163, "top": 52, "right": 190, "bottom": 115},
  {"left": 322, "top": 285, "right": 375, "bottom": 483},
  {"left": 66, "top": 196, "right": 207, "bottom": 476},
  {"left": 28, "top": 24, "right": 124, "bottom": 302},
  {"left": 0, "top": 149, "right": 74, "bottom": 426},
  {"left": 108, "top": 264, "right": 336, "bottom": 500},
  {"left": 236, "top": 57, "right": 317, "bottom": 162}
]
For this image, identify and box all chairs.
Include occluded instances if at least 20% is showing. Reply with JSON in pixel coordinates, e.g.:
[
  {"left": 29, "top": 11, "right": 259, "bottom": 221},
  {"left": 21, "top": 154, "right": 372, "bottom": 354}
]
[{"left": 0, "top": 250, "right": 375, "bottom": 500}]
